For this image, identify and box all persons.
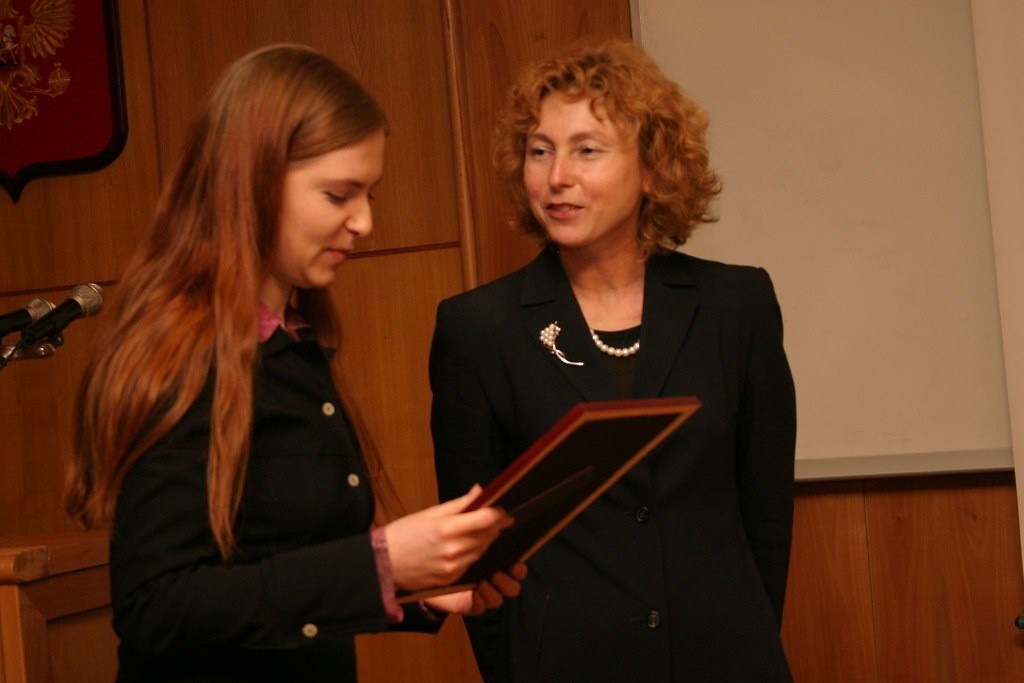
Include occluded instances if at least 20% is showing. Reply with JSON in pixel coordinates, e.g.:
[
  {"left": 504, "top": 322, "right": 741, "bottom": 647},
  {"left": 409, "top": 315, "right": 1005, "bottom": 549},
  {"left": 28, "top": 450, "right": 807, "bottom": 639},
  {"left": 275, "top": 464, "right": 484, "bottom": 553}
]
[
  {"left": 67, "top": 45, "right": 527, "bottom": 683},
  {"left": 428, "top": 41, "right": 796, "bottom": 683}
]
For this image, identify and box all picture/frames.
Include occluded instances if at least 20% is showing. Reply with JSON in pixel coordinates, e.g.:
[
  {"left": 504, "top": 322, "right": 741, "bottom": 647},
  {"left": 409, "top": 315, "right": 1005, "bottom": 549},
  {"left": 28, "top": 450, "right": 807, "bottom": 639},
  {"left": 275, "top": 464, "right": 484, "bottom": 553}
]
[{"left": 393, "top": 392, "right": 705, "bottom": 604}]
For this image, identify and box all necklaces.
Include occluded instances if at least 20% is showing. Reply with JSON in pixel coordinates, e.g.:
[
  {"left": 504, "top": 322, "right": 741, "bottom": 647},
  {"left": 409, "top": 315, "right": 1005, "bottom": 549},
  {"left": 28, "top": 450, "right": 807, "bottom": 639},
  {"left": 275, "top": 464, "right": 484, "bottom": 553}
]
[{"left": 589, "top": 329, "right": 638, "bottom": 356}]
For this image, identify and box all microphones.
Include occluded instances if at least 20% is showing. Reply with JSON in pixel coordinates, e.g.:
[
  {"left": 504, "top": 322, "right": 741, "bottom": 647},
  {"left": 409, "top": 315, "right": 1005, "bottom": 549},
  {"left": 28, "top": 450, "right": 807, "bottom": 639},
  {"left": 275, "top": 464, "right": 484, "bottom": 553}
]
[{"left": 0, "top": 283, "right": 106, "bottom": 348}]
